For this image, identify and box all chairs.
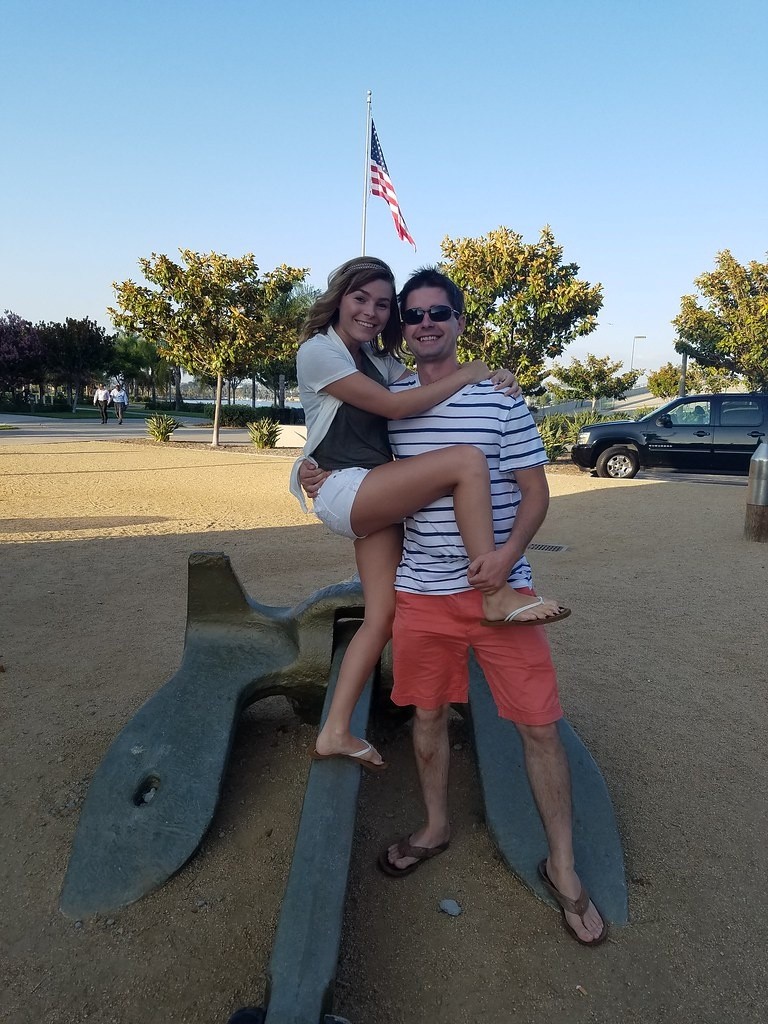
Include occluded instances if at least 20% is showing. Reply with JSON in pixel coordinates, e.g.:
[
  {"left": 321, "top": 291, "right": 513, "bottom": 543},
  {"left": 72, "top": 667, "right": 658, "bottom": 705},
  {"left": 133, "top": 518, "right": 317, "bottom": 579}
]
[{"left": 695, "top": 406, "right": 705, "bottom": 424}]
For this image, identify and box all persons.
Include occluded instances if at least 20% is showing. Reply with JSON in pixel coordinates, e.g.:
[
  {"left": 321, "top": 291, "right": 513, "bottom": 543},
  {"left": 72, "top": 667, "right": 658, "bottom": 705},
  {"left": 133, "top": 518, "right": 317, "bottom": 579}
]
[
  {"left": 94, "top": 382, "right": 110, "bottom": 424},
  {"left": 369, "top": 120, "right": 417, "bottom": 252},
  {"left": 110, "top": 385, "right": 128, "bottom": 424},
  {"left": 300, "top": 271, "right": 609, "bottom": 948},
  {"left": 290, "top": 257, "right": 571, "bottom": 774}
]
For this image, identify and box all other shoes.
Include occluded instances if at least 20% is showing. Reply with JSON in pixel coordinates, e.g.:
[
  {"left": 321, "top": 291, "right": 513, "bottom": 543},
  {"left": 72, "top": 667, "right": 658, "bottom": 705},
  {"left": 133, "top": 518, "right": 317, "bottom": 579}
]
[
  {"left": 117, "top": 420, "right": 123, "bottom": 425},
  {"left": 101, "top": 420, "right": 108, "bottom": 425}
]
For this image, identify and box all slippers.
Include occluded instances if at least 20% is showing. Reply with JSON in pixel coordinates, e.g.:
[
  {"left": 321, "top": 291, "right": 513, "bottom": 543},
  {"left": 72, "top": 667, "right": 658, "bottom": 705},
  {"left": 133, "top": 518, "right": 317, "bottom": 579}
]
[
  {"left": 536, "top": 858, "right": 609, "bottom": 948},
  {"left": 376, "top": 831, "right": 451, "bottom": 879},
  {"left": 479, "top": 595, "right": 572, "bottom": 627},
  {"left": 306, "top": 736, "right": 385, "bottom": 770}
]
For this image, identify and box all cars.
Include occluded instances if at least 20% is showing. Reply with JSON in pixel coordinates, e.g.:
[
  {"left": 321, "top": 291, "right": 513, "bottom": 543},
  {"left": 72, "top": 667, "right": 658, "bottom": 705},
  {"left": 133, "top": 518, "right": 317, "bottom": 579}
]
[{"left": 571, "top": 392, "right": 768, "bottom": 479}]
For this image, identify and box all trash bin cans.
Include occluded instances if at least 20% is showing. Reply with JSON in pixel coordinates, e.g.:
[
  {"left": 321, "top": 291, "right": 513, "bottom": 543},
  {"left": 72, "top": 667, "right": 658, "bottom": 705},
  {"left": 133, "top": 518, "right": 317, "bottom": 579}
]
[{"left": 742, "top": 442, "right": 768, "bottom": 541}]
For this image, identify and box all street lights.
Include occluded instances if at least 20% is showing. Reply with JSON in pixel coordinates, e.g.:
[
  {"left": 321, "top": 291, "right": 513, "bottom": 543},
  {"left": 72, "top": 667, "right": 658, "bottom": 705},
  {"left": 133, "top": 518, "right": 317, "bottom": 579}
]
[{"left": 631, "top": 336, "right": 646, "bottom": 371}]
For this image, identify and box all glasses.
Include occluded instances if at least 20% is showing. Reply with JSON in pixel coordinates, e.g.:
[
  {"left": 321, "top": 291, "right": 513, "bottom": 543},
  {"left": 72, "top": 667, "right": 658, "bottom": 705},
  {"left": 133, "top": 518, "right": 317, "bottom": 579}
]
[{"left": 401, "top": 304, "right": 461, "bottom": 326}]
